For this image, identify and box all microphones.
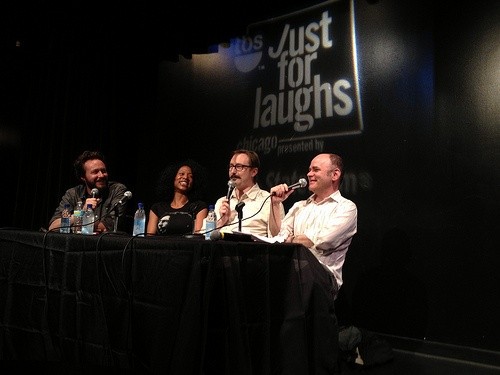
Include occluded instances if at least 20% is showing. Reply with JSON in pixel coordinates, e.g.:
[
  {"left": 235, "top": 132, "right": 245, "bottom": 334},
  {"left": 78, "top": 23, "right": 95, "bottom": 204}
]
[
  {"left": 90, "top": 188, "right": 98, "bottom": 198},
  {"left": 271, "top": 178, "right": 307, "bottom": 196},
  {"left": 210, "top": 230, "right": 251, "bottom": 241},
  {"left": 223, "top": 180, "right": 235, "bottom": 215},
  {"left": 113, "top": 191, "right": 132, "bottom": 210}
]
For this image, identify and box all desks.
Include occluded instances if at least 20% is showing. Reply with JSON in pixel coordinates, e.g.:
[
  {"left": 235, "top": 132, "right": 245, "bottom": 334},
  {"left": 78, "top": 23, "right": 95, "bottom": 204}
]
[{"left": 0, "top": 229, "right": 338, "bottom": 375}]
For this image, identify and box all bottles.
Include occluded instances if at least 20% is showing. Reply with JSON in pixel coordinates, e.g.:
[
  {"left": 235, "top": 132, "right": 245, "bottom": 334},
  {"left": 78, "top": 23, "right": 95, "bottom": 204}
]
[
  {"left": 72, "top": 201, "right": 83, "bottom": 234},
  {"left": 60, "top": 204, "right": 71, "bottom": 233},
  {"left": 133, "top": 203, "right": 145, "bottom": 237},
  {"left": 84, "top": 204, "right": 94, "bottom": 232},
  {"left": 206, "top": 205, "right": 217, "bottom": 240}
]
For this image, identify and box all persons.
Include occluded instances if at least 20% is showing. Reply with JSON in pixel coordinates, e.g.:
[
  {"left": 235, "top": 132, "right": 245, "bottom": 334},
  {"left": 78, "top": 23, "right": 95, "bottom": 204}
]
[
  {"left": 48, "top": 150, "right": 138, "bottom": 235},
  {"left": 199, "top": 149, "right": 285, "bottom": 238},
  {"left": 269, "top": 154, "right": 358, "bottom": 301},
  {"left": 147, "top": 161, "right": 208, "bottom": 237}
]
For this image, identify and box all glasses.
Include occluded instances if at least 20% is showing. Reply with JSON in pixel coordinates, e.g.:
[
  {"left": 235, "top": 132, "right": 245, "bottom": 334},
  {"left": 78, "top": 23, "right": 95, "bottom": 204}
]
[{"left": 227, "top": 164, "right": 257, "bottom": 171}]
